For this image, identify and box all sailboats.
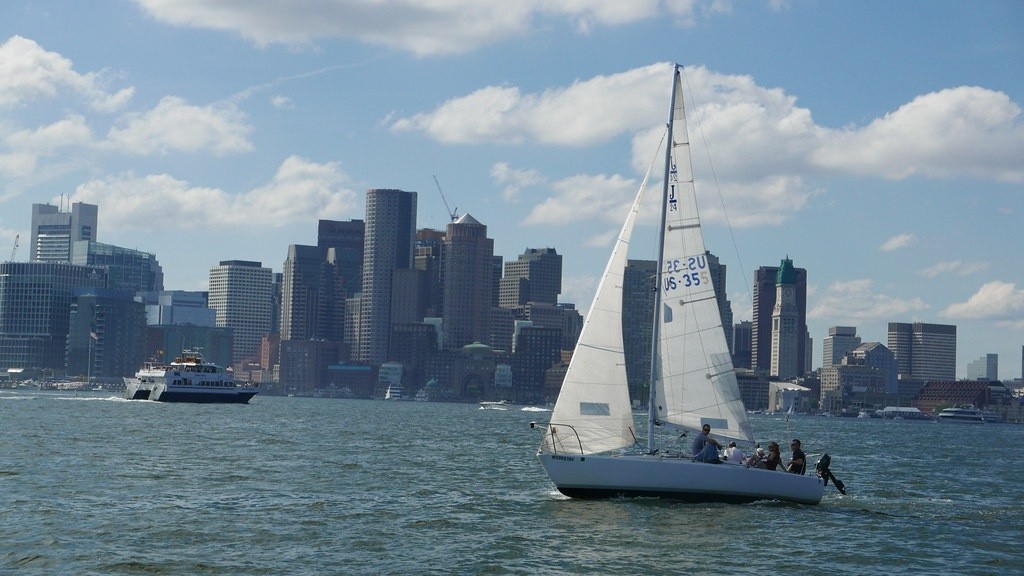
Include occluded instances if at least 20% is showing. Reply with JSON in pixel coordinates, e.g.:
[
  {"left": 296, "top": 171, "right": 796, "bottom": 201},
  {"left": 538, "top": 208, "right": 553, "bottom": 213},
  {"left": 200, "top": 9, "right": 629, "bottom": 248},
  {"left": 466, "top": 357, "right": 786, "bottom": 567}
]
[{"left": 530, "top": 62, "right": 828, "bottom": 507}]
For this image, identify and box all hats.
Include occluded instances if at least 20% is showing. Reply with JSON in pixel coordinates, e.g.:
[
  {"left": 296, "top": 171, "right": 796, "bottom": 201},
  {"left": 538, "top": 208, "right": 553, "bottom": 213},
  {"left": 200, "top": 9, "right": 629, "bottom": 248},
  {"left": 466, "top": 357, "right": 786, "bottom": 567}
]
[{"left": 729, "top": 441, "right": 736, "bottom": 445}]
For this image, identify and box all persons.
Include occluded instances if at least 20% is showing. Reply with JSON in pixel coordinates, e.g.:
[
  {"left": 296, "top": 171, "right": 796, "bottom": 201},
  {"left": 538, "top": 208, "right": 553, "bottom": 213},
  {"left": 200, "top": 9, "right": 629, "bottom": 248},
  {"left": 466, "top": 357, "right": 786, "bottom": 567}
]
[
  {"left": 787, "top": 439, "right": 806, "bottom": 475},
  {"left": 693, "top": 425, "right": 724, "bottom": 465},
  {"left": 723, "top": 440, "right": 744, "bottom": 464},
  {"left": 171, "top": 367, "right": 216, "bottom": 373},
  {"left": 745, "top": 442, "right": 787, "bottom": 472},
  {"left": 243, "top": 381, "right": 258, "bottom": 388}
]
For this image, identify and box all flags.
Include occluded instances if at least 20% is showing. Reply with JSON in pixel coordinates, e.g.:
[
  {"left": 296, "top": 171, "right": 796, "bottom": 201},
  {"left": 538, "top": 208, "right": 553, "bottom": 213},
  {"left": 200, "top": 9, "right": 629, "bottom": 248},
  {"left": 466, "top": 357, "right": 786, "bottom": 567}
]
[
  {"left": 226, "top": 365, "right": 233, "bottom": 372},
  {"left": 90, "top": 329, "right": 99, "bottom": 340}
]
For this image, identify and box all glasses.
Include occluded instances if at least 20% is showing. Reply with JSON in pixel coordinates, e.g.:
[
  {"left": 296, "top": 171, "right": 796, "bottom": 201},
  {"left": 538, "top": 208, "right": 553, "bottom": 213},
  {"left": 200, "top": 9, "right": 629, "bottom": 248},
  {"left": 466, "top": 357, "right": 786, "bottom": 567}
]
[{"left": 703, "top": 429, "right": 710, "bottom": 432}]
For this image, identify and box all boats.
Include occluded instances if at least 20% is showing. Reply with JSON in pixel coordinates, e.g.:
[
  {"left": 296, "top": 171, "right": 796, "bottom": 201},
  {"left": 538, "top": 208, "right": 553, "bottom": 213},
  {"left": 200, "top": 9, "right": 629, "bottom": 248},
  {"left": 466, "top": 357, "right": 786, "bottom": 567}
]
[
  {"left": 938, "top": 406, "right": 987, "bottom": 424},
  {"left": 122, "top": 343, "right": 262, "bottom": 404}
]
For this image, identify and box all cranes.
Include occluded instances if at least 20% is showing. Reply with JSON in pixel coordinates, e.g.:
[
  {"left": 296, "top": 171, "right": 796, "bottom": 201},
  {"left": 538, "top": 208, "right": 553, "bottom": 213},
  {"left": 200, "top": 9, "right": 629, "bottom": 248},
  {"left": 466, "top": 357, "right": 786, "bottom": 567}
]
[{"left": 11, "top": 233, "right": 20, "bottom": 262}]
